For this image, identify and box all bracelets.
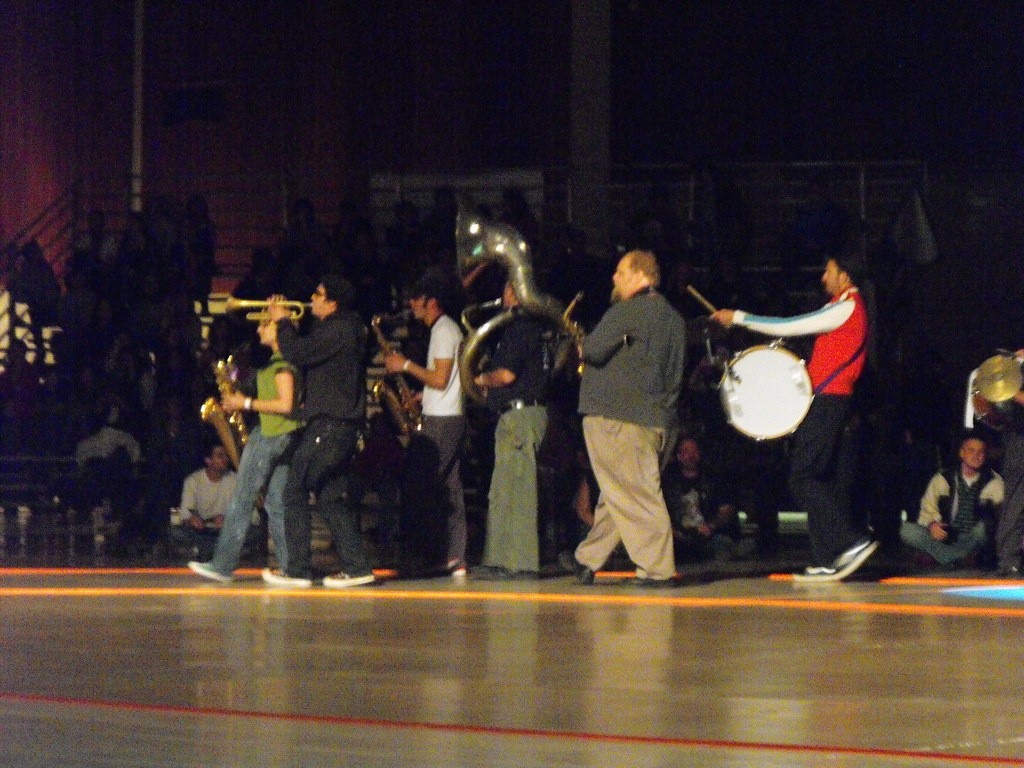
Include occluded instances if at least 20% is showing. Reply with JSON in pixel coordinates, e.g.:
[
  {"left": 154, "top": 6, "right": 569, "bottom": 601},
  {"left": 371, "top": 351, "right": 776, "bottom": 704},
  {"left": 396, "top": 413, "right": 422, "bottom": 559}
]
[
  {"left": 403, "top": 359, "right": 412, "bottom": 372},
  {"left": 479, "top": 372, "right": 484, "bottom": 386},
  {"left": 244, "top": 397, "right": 253, "bottom": 411}
]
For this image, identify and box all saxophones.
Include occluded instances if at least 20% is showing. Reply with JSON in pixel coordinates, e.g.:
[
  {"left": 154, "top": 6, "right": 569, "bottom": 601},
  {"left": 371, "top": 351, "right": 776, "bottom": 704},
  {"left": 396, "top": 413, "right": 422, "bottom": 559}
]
[
  {"left": 371, "top": 310, "right": 423, "bottom": 432},
  {"left": 202, "top": 352, "right": 249, "bottom": 470}
]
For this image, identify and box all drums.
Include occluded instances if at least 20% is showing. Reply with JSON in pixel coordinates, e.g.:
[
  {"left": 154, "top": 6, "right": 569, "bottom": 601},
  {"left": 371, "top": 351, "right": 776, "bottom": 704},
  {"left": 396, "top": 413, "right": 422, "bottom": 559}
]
[{"left": 717, "top": 340, "right": 813, "bottom": 442}]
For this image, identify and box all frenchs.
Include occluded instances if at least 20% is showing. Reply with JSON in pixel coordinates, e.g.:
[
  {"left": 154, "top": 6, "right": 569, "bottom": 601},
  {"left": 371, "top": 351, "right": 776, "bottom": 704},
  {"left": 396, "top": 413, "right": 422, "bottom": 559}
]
[{"left": 455, "top": 209, "right": 586, "bottom": 405}]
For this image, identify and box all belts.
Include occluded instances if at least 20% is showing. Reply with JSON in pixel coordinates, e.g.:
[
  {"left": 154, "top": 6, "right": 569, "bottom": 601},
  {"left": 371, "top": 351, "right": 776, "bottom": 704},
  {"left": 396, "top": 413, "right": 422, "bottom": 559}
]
[{"left": 499, "top": 399, "right": 546, "bottom": 415}]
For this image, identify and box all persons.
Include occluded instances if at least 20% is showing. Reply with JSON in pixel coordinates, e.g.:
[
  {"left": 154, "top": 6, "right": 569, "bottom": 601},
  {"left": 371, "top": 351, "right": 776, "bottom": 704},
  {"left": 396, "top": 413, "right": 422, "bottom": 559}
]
[
  {"left": 707, "top": 258, "right": 881, "bottom": 580},
  {"left": 386, "top": 279, "right": 467, "bottom": 580},
  {"left": 188, "top": 307, "right": 306, "bottom": 584},
  {"left": 560, "top": 249, "right": 687, "bottom": 589},
  {"left": 0, "top": 174, "right": 1024, "bottom": 569},
  {"left": 472, "top": 279, "right": 553, "bottom": 580},
  {"left": 261, "top": 273, "right": 375, "bottom": 588},
  {"left": 995, "top": 347, "right": 1024, "bottom": 578}
]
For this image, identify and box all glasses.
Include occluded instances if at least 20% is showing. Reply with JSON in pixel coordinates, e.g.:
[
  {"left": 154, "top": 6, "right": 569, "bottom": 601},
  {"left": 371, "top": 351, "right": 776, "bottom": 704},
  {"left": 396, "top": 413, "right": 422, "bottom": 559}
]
[{"left": 313, "top": 288, "right": 323, "bottom": 298}]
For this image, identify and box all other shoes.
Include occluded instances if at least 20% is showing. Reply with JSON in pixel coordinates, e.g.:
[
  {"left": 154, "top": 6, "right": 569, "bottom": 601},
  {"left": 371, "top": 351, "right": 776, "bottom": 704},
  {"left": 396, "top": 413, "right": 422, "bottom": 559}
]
[
  {"left": 437, "top": 557, "right": 467, "bottom": 576},
  {"left": 792, "top": 567, "right": 838, "bottom": 585},
  {"left": 557, "top": 549, "right": 595, "bottom": 585},
  {"left": 829, "top": 536, "right": 880, "bottom": 580},
  {"left": 620, "top": 574, "right": 674, "bottom": 589},
  {"left": 262, "top": 567, "right": 312, "bottom": 589},
  {"left": 185, "top": 560, "right": 232, "bottom": 584},
  {"left": 372, "top": 566, "right": 409, "bottom": 581},
  {"left": 323, "top": 569, "right": 376, "bottom": 587}
]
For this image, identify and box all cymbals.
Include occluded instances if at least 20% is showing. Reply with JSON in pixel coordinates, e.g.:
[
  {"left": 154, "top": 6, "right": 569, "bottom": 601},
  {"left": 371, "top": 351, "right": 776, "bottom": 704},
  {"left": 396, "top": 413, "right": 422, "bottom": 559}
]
[{"left": 976, "top": 355, "right": 1022, "bottom": 402}]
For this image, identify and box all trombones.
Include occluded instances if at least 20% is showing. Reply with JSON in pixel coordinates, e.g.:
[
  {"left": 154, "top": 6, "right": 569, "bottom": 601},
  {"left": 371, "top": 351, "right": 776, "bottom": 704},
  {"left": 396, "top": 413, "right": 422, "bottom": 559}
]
[{"left": 208, "top": 289, "right": 314, "bottom": 323}]
